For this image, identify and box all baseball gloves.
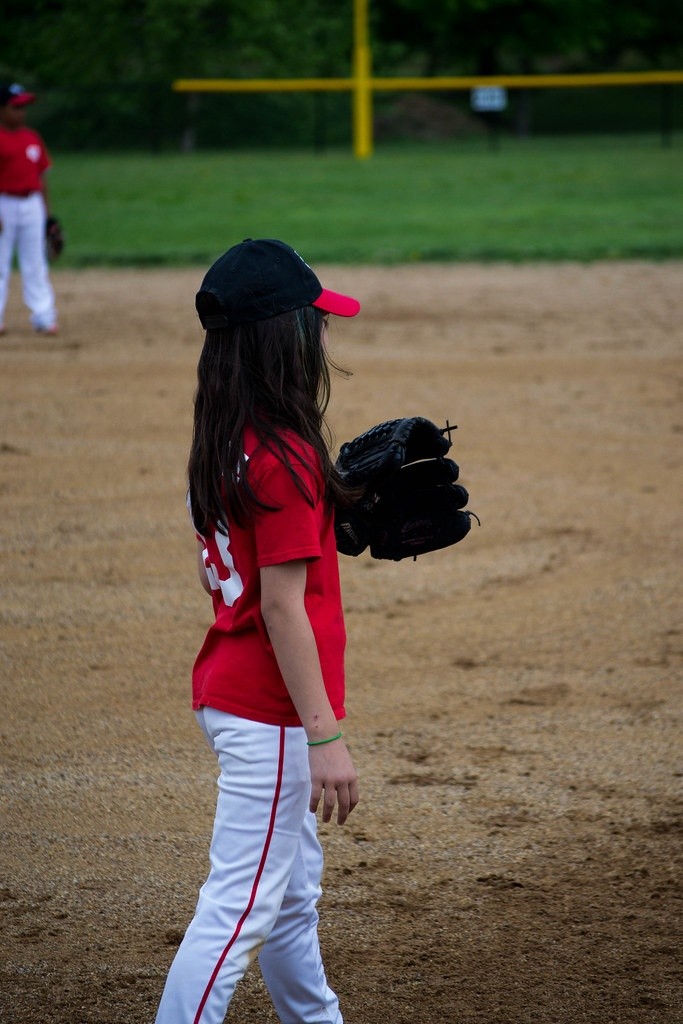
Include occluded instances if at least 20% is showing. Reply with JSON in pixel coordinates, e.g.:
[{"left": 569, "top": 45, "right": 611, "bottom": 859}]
[{"left": 332, "top": 415, "right": 473, "bottom": 563}]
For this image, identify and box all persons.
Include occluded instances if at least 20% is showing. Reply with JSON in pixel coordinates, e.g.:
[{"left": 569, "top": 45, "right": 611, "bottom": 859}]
[
  {"left": 154, "top": 242, "right": 471, "bottom": 1024},
  {"left": 0, "top": 85, "right": 60, "bottom": 335}
]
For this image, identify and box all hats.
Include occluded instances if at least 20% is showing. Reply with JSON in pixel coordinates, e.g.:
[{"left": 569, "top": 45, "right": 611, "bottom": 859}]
[
  {"left": 0, "top": 80, "right": 38, "bottom": 108},
  {"left": 195, "top": 239, "right": 360, "bottom": 329}
]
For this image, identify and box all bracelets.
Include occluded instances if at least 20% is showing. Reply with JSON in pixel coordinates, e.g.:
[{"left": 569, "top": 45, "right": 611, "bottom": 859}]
[{"left": 307, "top": 731, "right": 341, "bottom": 746}]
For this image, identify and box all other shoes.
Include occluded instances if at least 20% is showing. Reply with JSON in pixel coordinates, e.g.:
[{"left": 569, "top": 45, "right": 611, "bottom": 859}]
[{"left": 36, "top": 323, "right": 60, "bottom": 334}]
